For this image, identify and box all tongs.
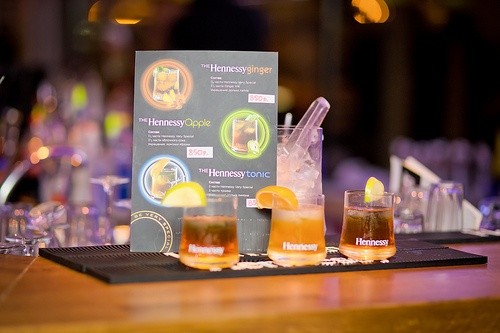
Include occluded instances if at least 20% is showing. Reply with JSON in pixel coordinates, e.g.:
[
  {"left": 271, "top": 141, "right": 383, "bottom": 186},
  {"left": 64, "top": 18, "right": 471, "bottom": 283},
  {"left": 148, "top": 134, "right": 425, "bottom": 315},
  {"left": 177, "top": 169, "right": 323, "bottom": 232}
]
[{"left": 281, "top": 95, "right": 330, "bottom": 172}]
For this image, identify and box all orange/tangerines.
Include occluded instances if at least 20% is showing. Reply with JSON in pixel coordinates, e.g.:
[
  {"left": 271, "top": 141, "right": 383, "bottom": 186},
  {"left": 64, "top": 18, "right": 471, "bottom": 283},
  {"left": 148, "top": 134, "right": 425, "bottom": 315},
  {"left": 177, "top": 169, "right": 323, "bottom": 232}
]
[
  {"left": 364, "top": 177, "right": 384, "bottom": 203},
  {"left": 162, "top": 182, "right": 207, "bottom": 208},
  {"left": 255, "top": 186, "right": 298, "bottom": 210}
]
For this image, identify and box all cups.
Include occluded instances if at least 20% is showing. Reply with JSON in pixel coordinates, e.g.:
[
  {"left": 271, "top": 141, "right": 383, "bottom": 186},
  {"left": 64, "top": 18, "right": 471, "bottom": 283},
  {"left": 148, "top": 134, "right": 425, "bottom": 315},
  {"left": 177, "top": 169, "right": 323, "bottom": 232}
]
[
  {"left": 393, "top": 189, "right": 424, "bottom": 235},
  {"left": 179, "top": 195, "right": 242, "bottom": 270},
  {"left": 424, "top": 177, "right": 464, "bottom": 233},
  {"left": 152, "top": 67, "right": 180, "bottom": 102},
  {"left": 231, "top": 119, "right": 258, "bottom": 152},
  {"left": 274, "top": 123, "right": 328, "bottom": 235},
  {"left": 2, "top": 189, "right": 115, "bottom": 257},
  {"left": 339, "top": 189, "right": 396, "bottom": 261},
  {"left": 266, "top": 191, "right": 326, "bottom": 268},
  {"left": 150, "top": 165, "right": 178, "bottom": 199}
]
